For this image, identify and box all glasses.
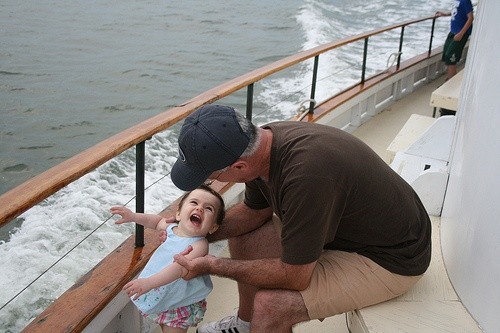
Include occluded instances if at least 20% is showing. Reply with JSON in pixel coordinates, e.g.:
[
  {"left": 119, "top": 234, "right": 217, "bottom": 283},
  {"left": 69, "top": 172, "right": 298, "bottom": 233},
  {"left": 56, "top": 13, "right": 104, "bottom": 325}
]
[{"left": 203, "top": 171, "right": 222, "bottom": 186}]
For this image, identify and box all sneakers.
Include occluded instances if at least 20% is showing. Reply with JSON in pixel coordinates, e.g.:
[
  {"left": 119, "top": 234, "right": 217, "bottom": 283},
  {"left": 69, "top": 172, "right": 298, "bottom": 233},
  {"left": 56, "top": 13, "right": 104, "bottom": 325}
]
[{"left": 195, "top": 307, "right": 251, "bottom": 333}]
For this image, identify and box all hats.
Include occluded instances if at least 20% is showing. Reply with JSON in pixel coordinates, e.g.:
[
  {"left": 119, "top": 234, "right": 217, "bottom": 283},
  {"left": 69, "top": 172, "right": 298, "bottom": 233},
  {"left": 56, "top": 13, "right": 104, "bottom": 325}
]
[{"left": 171, "top": 104, "right": 251, "bottom": 191}]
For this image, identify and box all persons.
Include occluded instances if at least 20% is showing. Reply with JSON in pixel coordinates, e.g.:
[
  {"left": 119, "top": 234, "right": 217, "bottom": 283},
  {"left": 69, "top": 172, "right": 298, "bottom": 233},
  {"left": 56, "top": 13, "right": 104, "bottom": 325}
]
[
  {"left": 435, "top": 0, "right": 474, "bottom": 80},
  {"left": 157, "top": 102, "right": 433, "bottom": 333},
  {"left": 110, "top": 181, "right": 225, "bottom": 333}
]
